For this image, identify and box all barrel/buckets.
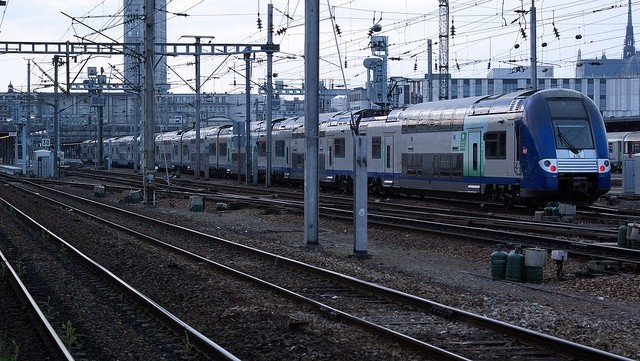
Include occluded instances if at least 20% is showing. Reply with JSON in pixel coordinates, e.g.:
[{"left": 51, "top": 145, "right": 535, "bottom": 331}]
[
  {"left": 523, "top": 266, "right": 545, "bottom": 284},
  {"left": 506, "top": 246, "right": 524, "bottom": 282},
  {"left": 489, "top": 244, "right": 509, "bottom": 280},
  {"left": 523, "top": 248, "right": 547, "bottom": 268}
]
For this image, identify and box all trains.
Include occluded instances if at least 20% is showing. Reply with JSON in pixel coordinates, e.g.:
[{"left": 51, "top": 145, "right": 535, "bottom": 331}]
[
  {"left": 80, "top": 88, "right": 612, "bottom": 211},
  {"left": 607, "top": 131, "right": 640, "bottom": 174}
]
